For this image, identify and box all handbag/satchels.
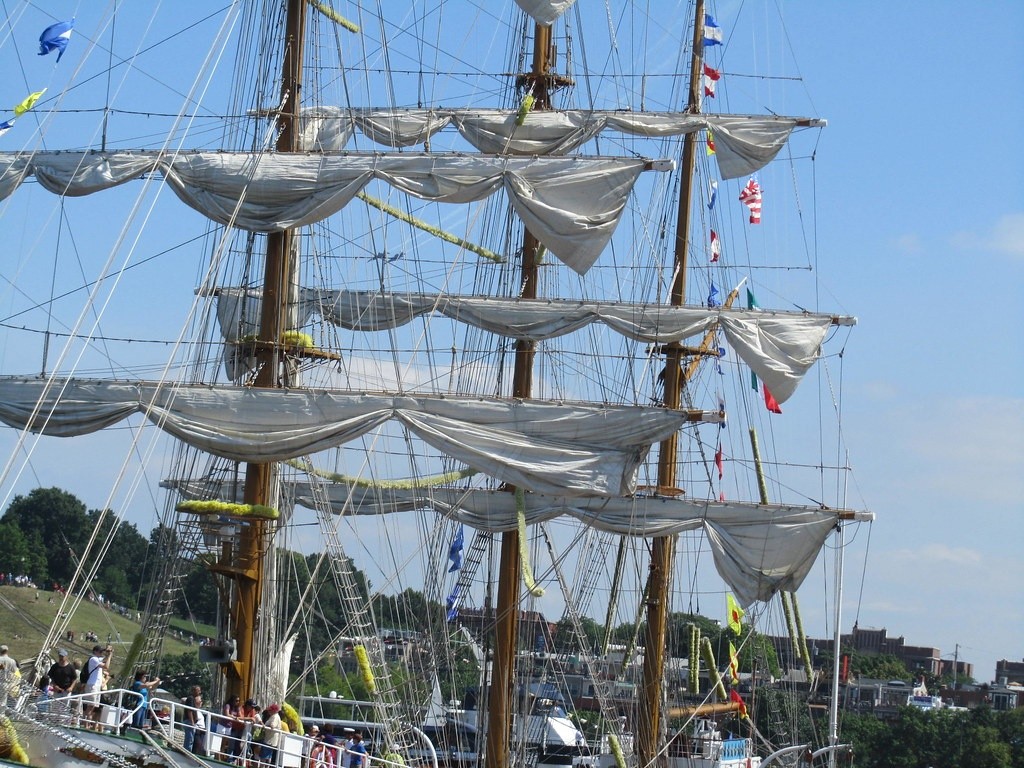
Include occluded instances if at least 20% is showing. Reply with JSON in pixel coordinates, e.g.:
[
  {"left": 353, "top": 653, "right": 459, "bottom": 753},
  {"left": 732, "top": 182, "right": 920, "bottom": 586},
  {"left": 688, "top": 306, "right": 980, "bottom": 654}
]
[{"left": 253, "top": 727, "right": 266, "bottom": 741}]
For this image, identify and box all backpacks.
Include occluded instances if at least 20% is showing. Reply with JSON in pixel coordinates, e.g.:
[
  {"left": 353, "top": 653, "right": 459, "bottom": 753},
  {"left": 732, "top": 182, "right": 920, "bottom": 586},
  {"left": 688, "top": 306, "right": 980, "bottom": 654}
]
[
  {"left": 79, "top": 656, "right": 99, "bottom": 683},
  {"left": 122, "top": 680, "right": 143, "bottom": 709}
]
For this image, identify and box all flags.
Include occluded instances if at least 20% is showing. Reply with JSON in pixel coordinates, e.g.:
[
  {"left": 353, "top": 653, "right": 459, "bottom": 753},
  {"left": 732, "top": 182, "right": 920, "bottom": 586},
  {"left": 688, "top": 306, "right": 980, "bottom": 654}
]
[
  {"left": 726, "top": 591, "right": 748, "bottom": 720},
  {"left": 704, "top": 63, "right": 721, "bottom": 98},
  {"left": 0, "top": 118, "right": 16, "bottom": 137},
  {"left": 739, "top": 177, "right": 764, "bottom": 224},
  {"left": 706, "top": 128, "right": 716, "bottom": 156},
  {"left": 707, "top": 178, "right": 717, "bottom": 209},
  {"left": 747, "top": 288, "right": 764, "bottom": 310},
  {"left": 750, "top": 369, "right": 758, "bottom": 392},
  {"left": 14, "top": 92, "right": 43, "bottom": 117},
  {"left": 445, "top": 526, "right": 465, "bottom": 622},
  {"left": 710, "top": 228, "right": 721, "bottom": 262},
  {"left": 763, "top": 383, "right": 782, "bottom": 414},
  {"left": 702, "top": 13, "right": 723, "bottom": 47},
  {"left": 708, "top": 282, "right": 726, "bottom": 501},
  {"left": 37, "top": 19, "right": 74, "bottom": 63}
]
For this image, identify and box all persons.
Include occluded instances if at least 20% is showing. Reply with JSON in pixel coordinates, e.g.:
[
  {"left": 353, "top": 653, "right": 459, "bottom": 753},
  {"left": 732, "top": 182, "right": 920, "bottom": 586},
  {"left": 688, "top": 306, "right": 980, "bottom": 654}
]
[
  {"left": 0, "top": 645, "right": 404, "bottom": 768},
  {"left": 0, "top": 571, "right": 213, "bottom": 647}
]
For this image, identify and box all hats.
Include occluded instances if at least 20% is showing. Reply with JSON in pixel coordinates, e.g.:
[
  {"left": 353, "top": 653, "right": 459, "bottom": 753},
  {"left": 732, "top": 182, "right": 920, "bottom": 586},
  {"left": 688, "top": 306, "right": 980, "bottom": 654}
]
[
  {"left": 390, "top": 744, "right": 401, "bottom": 751},
  {"left": 310, "top": 725, "right": 320, "bottom": 733},
  {"left": 59, "top": 650, "right": 69, "bottom": 657},
  {"left": 0, "top": 645, "right": 8, "bottom": 651},
  {"left": 352, "top": 734, "right": 362, "bottom": 741},
  {"left": 252, "top": 704, "right": 260, "bottom": 712},
  {"left": 266, "top": 704, "right": 279, "bottom": 712},
  {"left": 92, "top": 645, "right": 106, "bottom": 652}
]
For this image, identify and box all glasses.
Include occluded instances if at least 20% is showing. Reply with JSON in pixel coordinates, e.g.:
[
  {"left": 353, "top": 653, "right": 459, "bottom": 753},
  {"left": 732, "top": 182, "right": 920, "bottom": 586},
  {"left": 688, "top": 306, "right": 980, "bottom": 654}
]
[{"left": 235, "top": 700, "right": 240, "bottom": 703}]
